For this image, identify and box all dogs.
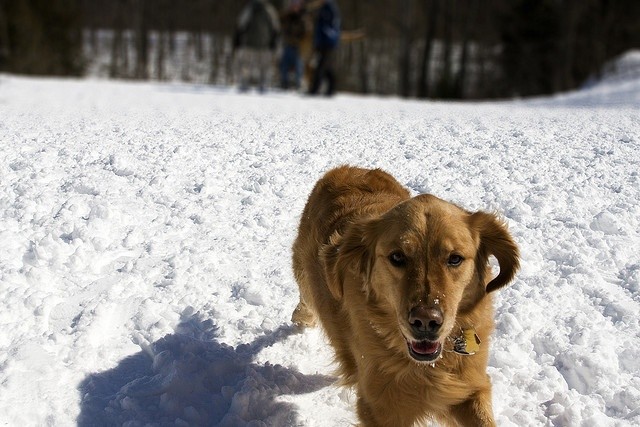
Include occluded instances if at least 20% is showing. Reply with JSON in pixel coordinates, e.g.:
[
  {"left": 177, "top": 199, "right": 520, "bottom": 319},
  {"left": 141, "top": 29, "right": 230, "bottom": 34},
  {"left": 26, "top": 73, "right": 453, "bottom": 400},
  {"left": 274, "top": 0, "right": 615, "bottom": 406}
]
[{"left": 291, "top": 164, "right": 521, "bottom": 426}]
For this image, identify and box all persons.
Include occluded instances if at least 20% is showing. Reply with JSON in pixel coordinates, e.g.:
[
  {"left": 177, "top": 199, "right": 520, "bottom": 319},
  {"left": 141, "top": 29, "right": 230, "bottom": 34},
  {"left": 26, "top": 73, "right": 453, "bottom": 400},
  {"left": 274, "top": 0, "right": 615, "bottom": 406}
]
[
  {"left": 308, "top": 0, "right": 345, "bottom": 96},
  {"left": 279, "top": 0, "right": 312, "bottom": 93},
  {"left": 232, "top": 0, "right": 279, "bottom": 95}
]
